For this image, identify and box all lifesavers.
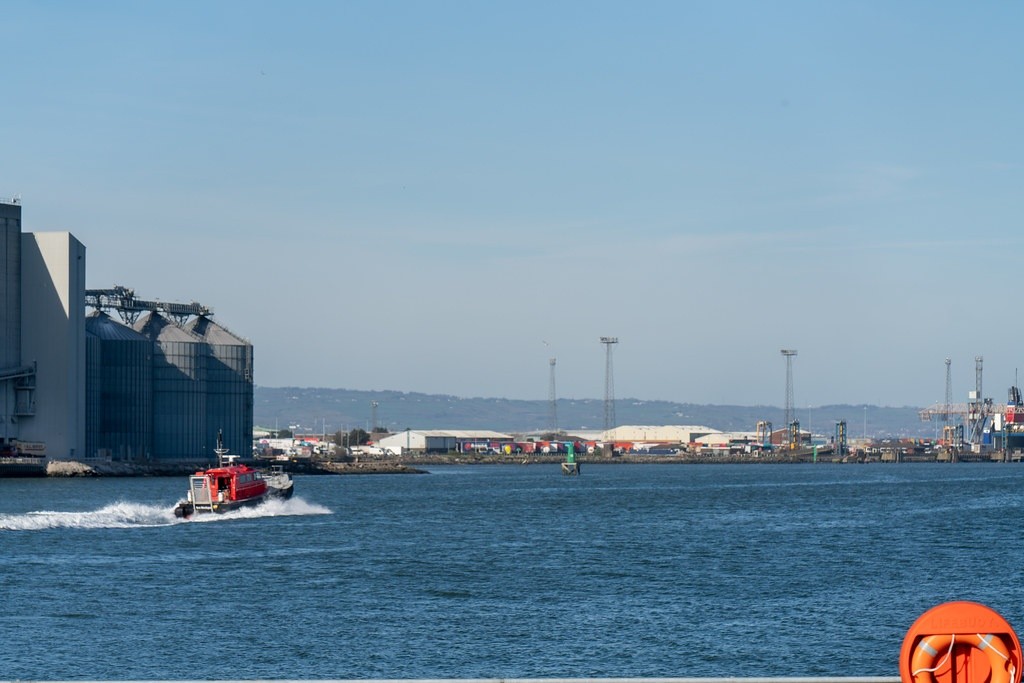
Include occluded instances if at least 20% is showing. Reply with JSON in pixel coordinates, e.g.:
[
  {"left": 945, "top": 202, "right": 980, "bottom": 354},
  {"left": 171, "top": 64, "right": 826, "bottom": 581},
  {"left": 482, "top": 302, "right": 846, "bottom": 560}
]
[{"left": 910, "top": 633, "right": 1016, "bottom": 683}]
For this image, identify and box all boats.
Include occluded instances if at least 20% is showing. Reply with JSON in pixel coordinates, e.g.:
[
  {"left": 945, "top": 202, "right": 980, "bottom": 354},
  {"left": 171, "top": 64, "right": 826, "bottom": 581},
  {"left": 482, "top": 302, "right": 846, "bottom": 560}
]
[{"left": 174, "top": 429, "right": 294, "bottom": 521}]
